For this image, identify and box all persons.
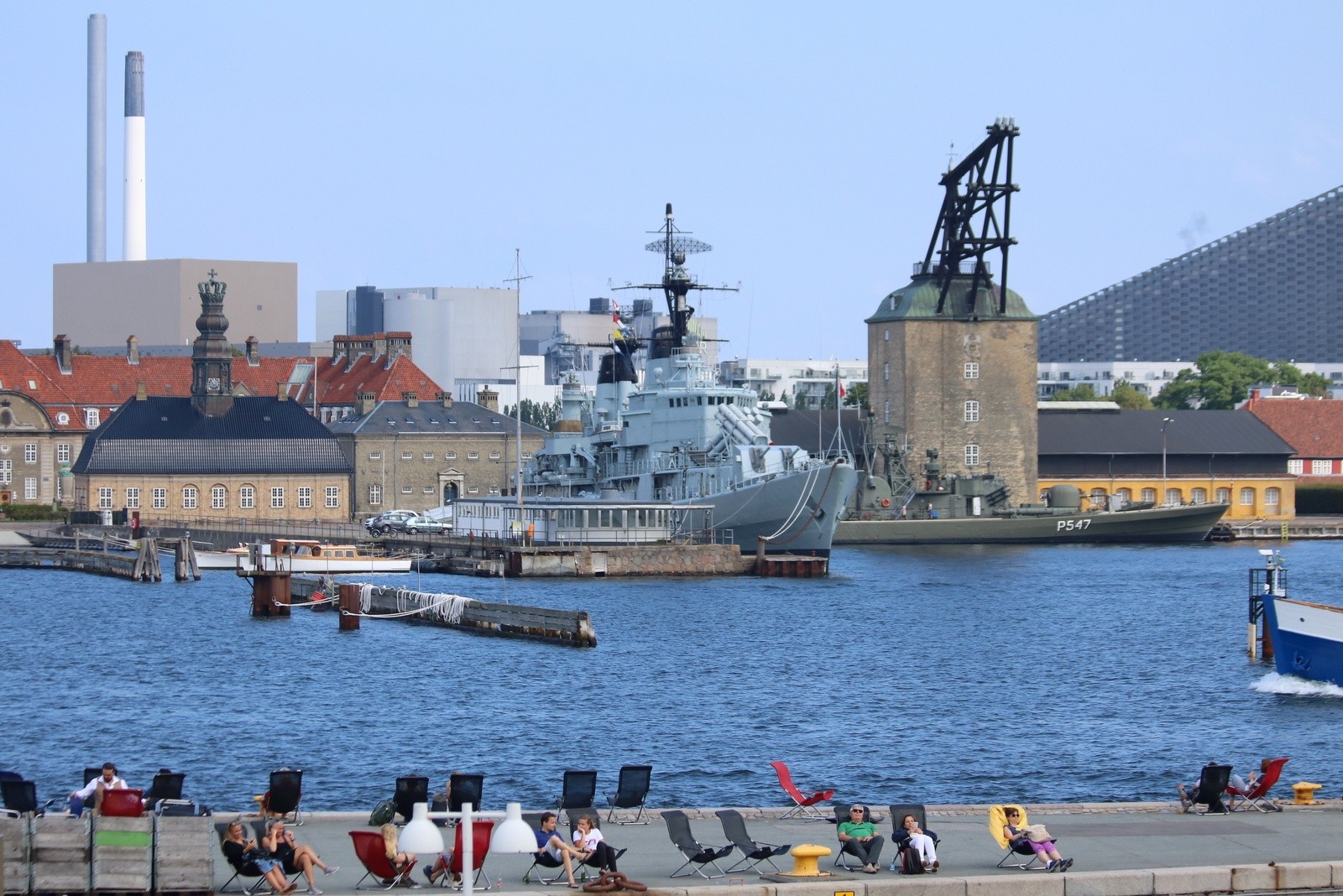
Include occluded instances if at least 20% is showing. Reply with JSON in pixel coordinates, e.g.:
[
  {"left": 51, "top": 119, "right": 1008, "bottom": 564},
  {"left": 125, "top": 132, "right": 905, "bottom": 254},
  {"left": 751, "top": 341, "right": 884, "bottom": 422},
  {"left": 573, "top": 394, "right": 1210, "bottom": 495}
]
[
  {"left": 892, "top": 815, "right": 940, "bottom": 873},
  {"left": 1224, "top": 758, "right": 1275, "bottom": 811},
  {"left": 261, "top": 819, "right": 340, "bottom": 895},
  {"left": 837, "top": 804, "right": 885, "bottom": 873},
  {"left": 221, "top": 821, "right": 298, "bottom": 896},
  {"left": 374, "top": 824, "right": 418, "bottom": 887},
  {"left": 531, "top": 811, "right": 595, "bottom": 888},
  {"left": 1002, "top": 806, "right": 1073, "bottom": 874},
  {"left": 253, "top": 768, "right": 302, "bottom": 819},
  {"left": 391, "top": 773, "right": 425, "bottom": 820},
  {"left": 1177, "top": 762, "right": 1221, "bottom": 812},
  {"left": 444, "top": 770, "right": 479, "bottom": 826},
  {"left": 424, "top": 818, "right": 488, "bottom": 887},
  {"left": 71, "top": 762, "right": 151, "bottom": 818},
  {"left": 573, "top": 814, "right": 625, "bottom": 891},
  {"left": 142, "top": 768, "right": 181, "bottom": 810}
]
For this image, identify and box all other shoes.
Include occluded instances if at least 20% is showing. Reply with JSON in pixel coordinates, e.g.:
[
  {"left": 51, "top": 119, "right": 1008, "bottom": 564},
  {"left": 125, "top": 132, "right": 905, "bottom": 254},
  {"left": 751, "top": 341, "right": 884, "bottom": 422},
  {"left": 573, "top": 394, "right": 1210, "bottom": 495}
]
[
  {"left": 382, "top": 878, "right": 398, "bottom": 886},
  {"left": 862, "top": 863, "right": 879, "bottom": 873},
  {"left": 399, "top": 877, "right": 417, "bottom": 886},
  {"left": 324, "top": 866, "right": 339, "bottom": 876},
  {"left": 931, "top": 860, "right": 939, "bottom": 872},
  {"left": 308, "top": 888, "right": 322, "bottom": 895},
  {"left": 921, "top": 860, "right": 926, "bottom": 867},
  {"left": 579, "top": 853, "right": 592, "bottom": 862},
  {"left": 567, "top": 883, "right": 578, "bottom": 888},
  {"left": 423, "top": 865, "right": 436, "bottom": 884},
  {"left": 599, "top": 871, "right": 605, "bottom": 875},
  {"left": 282, "top": 814, "right": 288, "bottom": 818},
  {"left": 278, "top": 883, "right": 297, "bottom": 895}
]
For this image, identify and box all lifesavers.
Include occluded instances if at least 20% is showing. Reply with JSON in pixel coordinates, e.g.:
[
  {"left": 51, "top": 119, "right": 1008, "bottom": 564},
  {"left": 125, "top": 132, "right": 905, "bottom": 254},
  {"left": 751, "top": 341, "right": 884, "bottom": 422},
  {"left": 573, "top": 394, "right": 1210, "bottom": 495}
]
[
  {"left": 924, "top": 479, "right": 933, "bottom": 490},
  {"left": 43, "top": 541, "right": 123, "bottom": 550},
  {"left": 881, "top": 498, "right": 890, "bottom": 508}
]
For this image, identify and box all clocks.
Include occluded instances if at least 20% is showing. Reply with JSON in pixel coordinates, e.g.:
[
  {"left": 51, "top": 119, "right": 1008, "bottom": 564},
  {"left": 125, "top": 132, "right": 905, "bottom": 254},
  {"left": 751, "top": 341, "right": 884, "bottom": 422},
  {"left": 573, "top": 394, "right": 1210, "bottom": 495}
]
[{"left": 208, "top": 377, "right": 220, "bottom": 391}]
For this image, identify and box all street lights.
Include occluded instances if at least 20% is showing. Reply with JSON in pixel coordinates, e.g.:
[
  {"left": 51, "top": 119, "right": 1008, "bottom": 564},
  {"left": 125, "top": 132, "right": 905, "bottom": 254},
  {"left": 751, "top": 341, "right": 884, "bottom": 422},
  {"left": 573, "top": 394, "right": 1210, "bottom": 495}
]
[{"left": 1160, "top": 417, "right": 1175, "bottom": 479}]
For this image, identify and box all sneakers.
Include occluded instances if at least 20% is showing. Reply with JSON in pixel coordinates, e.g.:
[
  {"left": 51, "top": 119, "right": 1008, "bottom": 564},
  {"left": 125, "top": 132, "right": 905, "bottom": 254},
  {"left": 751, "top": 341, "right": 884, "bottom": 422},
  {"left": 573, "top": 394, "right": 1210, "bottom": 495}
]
[
  {"left": 1059, "top": 858, "right": 1073, "bottom": 872},
  {"left": 1047, "top": 858, "right": 1061, "bottom": 873}
]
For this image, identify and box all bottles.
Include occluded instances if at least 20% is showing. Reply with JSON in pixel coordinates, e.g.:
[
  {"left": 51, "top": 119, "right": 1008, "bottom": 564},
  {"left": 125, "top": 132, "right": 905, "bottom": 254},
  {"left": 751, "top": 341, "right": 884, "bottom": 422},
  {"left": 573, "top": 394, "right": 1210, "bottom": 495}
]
[
  {"left": 525, "top": 871, "right": 529, "bottom": 885},
  {"left": 497, "top": 873, "right": 502, "bottom": 889},
  {"left": 890, "top": 854, "right": 895, "bottom": 871},
  {"left": 581, "top": 865, "right": 586, "bottom": 885}
]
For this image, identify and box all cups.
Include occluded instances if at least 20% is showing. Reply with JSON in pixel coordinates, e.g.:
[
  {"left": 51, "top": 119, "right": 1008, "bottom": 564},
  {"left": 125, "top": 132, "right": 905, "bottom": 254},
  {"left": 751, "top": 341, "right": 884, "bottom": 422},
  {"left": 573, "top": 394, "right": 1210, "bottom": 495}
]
[{"left": 913, "top": 822, "right": 919, "bottom": 828}]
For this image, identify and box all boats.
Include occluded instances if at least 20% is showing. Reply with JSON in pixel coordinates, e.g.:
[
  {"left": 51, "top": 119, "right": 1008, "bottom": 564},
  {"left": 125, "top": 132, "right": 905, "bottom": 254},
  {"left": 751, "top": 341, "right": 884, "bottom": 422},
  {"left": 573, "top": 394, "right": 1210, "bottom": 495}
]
[
  {"left": 190, "top": 538, "right": 413, "bottom": 572},
  {"left": 510, "top": 202, "right": 862, "bottom": 573},
  {"left": 818, "top": 363, "right": 1232, "bottom": 546},
  {"left": 1249, "top": 549, "right": 1343, "bottom": 695}
]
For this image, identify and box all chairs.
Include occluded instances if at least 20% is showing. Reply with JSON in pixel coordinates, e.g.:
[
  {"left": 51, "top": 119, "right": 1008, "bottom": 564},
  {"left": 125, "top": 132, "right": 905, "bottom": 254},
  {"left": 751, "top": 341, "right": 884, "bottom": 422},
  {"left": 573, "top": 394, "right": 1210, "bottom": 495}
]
[
  {"left": 0, "top": 768, "right": 210, "bottom": 818},
  {"left": 522, "top": 765, "right": 653, "bottom": 885},
  {"left": 771, "top": 761, "right": 834, "bottom": 821},
  {"left": 349, "top": 773, "right": 494, "bottom": 891},
  {"left": 890, "top": 804, "right": 940, "bottom": 871},
  {"left": 1181, "top": 758, "right": 1289, "bottom": 815},
  {"left": 661, "top": 810, "right": 735, "bottom": 880},
  {"left": 715, "top": 810, "right": 791, "bottom": 874},
  {"left": 215, "top": 769, "right": 318, "bottom": 896},
  {"left": 990, "top": 804, "right": 1057, "bottom": 871},
  {"left": 834, "top": 806, "right": 880, "bottom": 871}
]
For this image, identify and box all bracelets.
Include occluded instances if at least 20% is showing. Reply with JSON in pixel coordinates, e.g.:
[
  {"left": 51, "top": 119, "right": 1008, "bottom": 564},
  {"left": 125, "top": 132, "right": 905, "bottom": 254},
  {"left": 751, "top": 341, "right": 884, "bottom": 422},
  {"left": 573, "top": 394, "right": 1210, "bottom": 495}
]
[
  {"left": 291, "top": 838, "right": 295, "bottom": 842},
  {"left": 581, "top": 847, "right": 585, "bottom": 852}
]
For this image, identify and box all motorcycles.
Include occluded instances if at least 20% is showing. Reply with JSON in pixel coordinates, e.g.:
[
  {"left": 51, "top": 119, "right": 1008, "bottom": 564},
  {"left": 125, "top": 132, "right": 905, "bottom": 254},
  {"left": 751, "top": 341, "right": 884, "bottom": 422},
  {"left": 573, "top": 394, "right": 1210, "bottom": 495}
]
[{"left": 370, "top": 522, "right": 398, "bottom": 538}]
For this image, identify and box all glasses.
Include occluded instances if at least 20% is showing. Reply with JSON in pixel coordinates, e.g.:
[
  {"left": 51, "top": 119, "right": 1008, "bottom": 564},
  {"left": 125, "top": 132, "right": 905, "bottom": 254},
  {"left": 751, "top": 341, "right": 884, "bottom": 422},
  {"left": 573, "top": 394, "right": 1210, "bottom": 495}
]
[
  {"left": 852, "top": 809, "right": 862, "bottom": 813},
  {"left": 1011, "top": 814, "right": 1019, "bottom": 817},
  {"left": 228, "top": 820, "right": 240, "bottom": 832}
]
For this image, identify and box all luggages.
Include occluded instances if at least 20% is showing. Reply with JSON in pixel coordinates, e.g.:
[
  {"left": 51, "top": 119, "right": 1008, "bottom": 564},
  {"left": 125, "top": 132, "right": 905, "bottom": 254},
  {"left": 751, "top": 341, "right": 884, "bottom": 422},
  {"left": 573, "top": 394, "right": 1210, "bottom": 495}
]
[{"left": 429, "top": 791, "right": 448, "bottom": 826}]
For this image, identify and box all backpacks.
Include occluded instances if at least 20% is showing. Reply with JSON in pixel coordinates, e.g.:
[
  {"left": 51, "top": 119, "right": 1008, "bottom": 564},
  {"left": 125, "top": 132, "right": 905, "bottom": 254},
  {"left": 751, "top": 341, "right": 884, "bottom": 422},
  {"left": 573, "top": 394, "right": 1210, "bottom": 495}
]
[
  {"left": 1208, "top": 798, "right": 1228, "bottom": 813},
  {"left": 369, "top": 798, "right": 395, "bottom": 826},
  {"left": 899, "top": 845, "right": 925, "bottom": 874}
]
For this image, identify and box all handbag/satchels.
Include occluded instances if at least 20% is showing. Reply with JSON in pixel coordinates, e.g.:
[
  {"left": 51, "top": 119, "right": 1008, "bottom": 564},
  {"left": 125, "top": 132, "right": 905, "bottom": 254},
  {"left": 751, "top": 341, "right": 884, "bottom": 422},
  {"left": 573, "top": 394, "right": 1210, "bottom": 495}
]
[
  {"left": 1022, "top": 824, "right": 1051, "bottom": 843},
  {"left": 247, "top": 848, "right": 269, "bottom": 859}
]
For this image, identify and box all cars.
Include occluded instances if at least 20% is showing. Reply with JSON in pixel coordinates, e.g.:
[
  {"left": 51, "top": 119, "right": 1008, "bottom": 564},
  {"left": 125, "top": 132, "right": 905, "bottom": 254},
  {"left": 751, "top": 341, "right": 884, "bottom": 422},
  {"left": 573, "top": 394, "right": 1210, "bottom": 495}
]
[{"left": 364, "top": 510, "right": 453, "bottom": 535}]
